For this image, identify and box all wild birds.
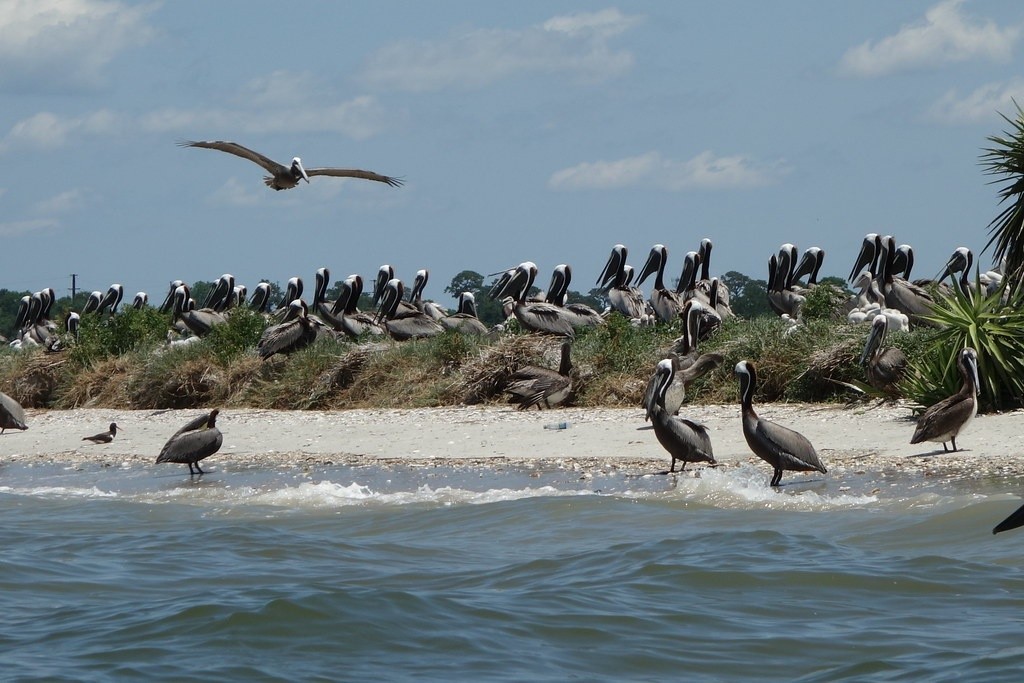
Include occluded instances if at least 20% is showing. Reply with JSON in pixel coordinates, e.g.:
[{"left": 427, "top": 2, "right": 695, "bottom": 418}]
[
  {"left": 174, "top": 138, "right": 406, "bottom": 191},
  {"left": 0, "top": 233, "right": 1016, "bottom": 486}
]
